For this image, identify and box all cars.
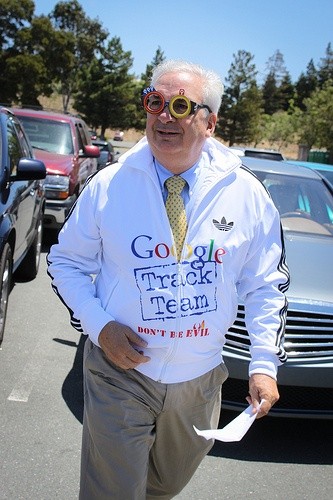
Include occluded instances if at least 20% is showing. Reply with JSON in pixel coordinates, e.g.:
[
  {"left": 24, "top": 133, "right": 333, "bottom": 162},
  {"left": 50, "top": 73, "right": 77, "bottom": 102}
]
[
  {"left": 209, "top": 156, "right": 333, "bottom": 419},
  {"left": 261, "top": 160, "right": 333, "bottom": 225},
  {"left": 9, "top": 108, "right": 101, "bottom": 229},
  {"left": 245, "top": 149, "right": 284, "bottom": 160},
  {"left": 113, "top": 134, "right": 123, "bottom": 142},
  {"left": 87, "top": 130, "right": 113, "bottom": 169},
  {"left": 0, "top": 105, "right": 46, "bottom": 345}
]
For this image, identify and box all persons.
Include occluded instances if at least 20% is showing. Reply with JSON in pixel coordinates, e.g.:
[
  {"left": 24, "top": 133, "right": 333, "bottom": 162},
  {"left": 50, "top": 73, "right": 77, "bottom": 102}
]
[{"left": 46, "top": 61, "right": 291, "bottom": 500}]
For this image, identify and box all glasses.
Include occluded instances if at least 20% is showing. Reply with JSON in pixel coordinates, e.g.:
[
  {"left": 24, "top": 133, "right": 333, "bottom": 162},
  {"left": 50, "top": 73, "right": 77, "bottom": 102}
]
[{"left": 142, "top": 90, "right": 213, "bottom": 118}]
